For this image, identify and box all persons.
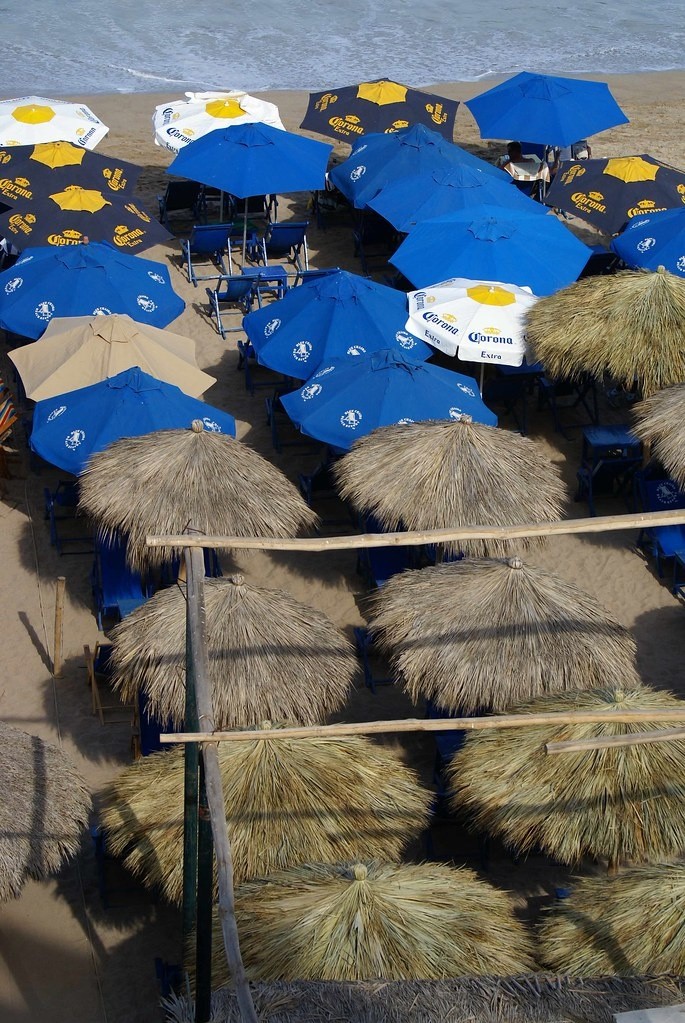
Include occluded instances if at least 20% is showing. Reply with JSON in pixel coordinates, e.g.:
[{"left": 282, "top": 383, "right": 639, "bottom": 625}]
[{"left": 500, "top": 141, "right": 535, "bottom": 185}]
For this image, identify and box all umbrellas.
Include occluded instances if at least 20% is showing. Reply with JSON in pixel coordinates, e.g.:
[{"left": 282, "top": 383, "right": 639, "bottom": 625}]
[
  {"left": 278, "top": 348, "right": 500, "bottom": 450},
  {"left": 181, "top": 854, "right": 538, "bottom": 991},
  {"left": 106, "top": 573, "right": 360, "bottom": 732},
  {"left": 79, "top": 418, "right": 319, "bottom": 569},
  {"left": 463, "top": 72, "right": 629, "bottom": 149},
  {"left": 7, "top": 313, "right": 238, "bottom": 478},
  {"left": 405, "top": 275, "right": 540, "bottom": 400},
  {"left": 330, "top": 416, "right": 568, "bottom": 558},
  {"left": 99, "top": 714, "right": 435, "bottom": 899},
  {"left": 366, "top": 556, "right": 645, "bottom": 706},
  {"left": 523, "top": 262, "right": 685, "bottom": 493},
  {"left": 151, "top": 88, "right": 334, "bottom": 267},
  {"left": 450, "top": 682, "right": 685, "bottom": 867},
  {"left": 544, "top": 153, "right": 685, "bottom": 276},
  {"left": 0, "top": 719, "right": 93, "bottom": 904},
  {"left": 300, "top": 76, "right": 551, "bottom": 237},
  {"left": 385, "top": 203, "right": 593, "bottom": 297},
  {"left": 0, "top": 96, "right": 187, "bottom": 338},
  {"left": 534, "top": 861, "right": 685, "bottom": 977},
  {"left": 241, "top": 267, "right": 434, "bottom": 380}
]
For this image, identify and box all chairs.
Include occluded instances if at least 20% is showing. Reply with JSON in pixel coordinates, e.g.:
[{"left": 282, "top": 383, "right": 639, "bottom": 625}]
[{"left": 2, "top": 140, "right": 685, "bottom": 1009}]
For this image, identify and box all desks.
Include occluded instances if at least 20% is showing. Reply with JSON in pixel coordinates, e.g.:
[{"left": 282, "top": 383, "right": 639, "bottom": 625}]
[
  {"left": 497, "top": 360, "right": 546, "bottom": 394},
  {"left": 580, "top": 425, "right": 641, "bottom": 469},
  {"left": 209, "top": 220, "right": 257, "bottom": 253},
  {"left": 240, "top": 264, "right": 288, "bottom": 305},
  {"left": 434, "top": 734, "right": 467, "bottom": 787},
  {"left": 673, "top": 549, "right": 685, "bottom": 596}
]
